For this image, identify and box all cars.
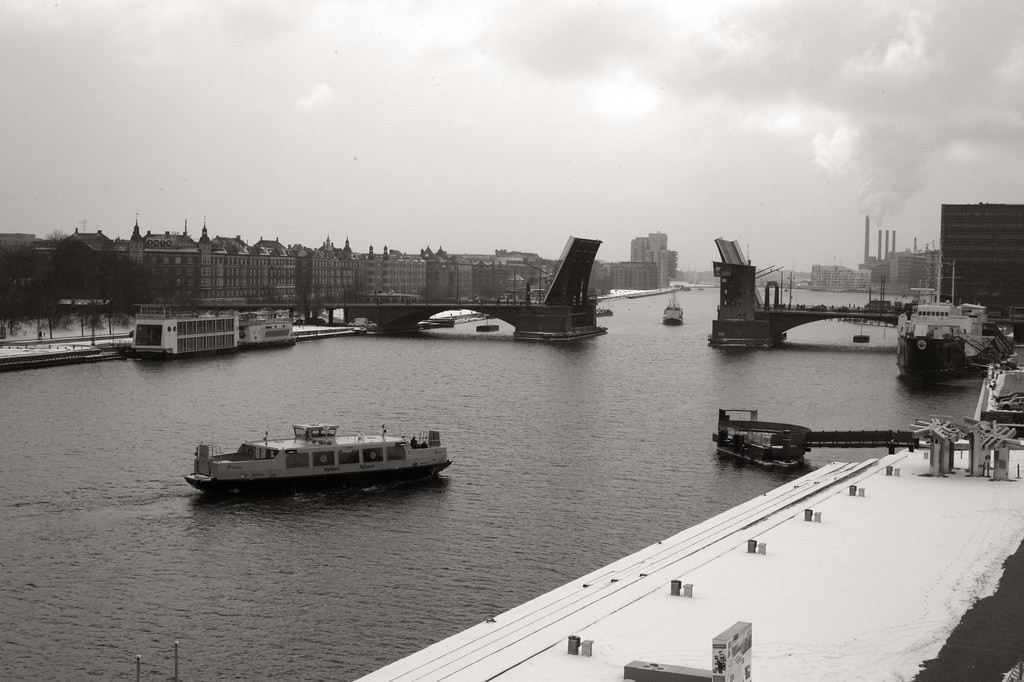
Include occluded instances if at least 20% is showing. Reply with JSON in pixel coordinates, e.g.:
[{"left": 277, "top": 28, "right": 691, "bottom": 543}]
[{"left": 806, "top": 305, "right": 825, "bottom": 312}]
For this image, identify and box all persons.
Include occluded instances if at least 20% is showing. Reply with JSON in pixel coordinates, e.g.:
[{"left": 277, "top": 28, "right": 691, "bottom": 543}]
[
  {"left": 39, "top": 331, "right": 42, "bottom": 339},
  {"left": 411, "top": 437, "right": 429, "bottom": 449}
]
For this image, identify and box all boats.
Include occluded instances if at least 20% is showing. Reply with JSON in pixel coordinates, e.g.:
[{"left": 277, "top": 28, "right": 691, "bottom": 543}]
[
  {"left": 662, "top": 291, "right": 684, "bottom": 326},
  {"left": 975, "top": 359, "right": 1024, "bottom": 441},
  {"left": 128, "top": 304, "right": 299, "bottom": 362},
  {"left": 183, "top": 421, "right": 453, "bottom": 504},
  {"left": 896, "top": 251, "right": 986, "bottom": 383}
]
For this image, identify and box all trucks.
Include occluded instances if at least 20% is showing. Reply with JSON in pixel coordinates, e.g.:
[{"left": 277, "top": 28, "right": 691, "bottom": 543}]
[{"left": 348, "top": 318, "right": 368, "bottom": 327}]
